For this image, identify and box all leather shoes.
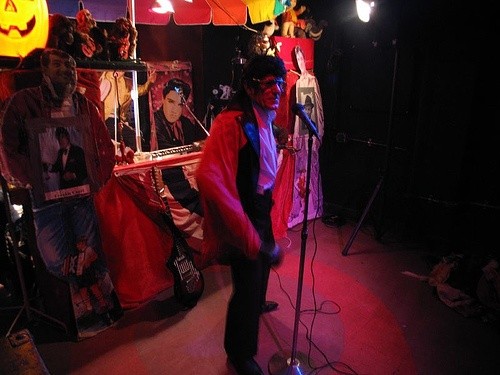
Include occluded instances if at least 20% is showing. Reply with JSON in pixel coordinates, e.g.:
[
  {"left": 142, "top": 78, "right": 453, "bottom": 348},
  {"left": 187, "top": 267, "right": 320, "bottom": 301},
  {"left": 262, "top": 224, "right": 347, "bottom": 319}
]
[
  {"left": 228, "top": 356, "right": 264, "bottom": 375},
  {"left": 265, "top": 301, "right": 279, "bottom": 312}
]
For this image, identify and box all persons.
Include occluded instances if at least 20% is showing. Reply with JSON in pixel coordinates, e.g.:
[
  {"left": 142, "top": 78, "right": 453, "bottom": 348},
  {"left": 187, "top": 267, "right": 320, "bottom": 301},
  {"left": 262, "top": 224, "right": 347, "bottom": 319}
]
[
  {"left": 195, "top": 54, "right": 287, "bottom": 375},
  {"left": 101, "top": 69, "right": 157, "bottom": 141},
  {"left": 288, "top": 46, "right": 324, "bottom": 222},
  {"left": 301, "top": 95, "right": 315, "bottom": 130},
  {"left": 42, "top": 127, "right": 88, "bottom": 188},
  {"left": 3, "top": 49, "right": 123, "bottom": 332}
]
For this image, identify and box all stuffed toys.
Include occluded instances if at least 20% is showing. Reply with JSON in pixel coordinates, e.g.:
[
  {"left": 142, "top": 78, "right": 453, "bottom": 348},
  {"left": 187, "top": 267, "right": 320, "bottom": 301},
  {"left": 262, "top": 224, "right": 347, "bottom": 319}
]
[
  {"left": 262, "top": 0, "right": 322, "bottom": 41},
  {"left": 48, "top": 10, "right": 138, "bottom": 62}
]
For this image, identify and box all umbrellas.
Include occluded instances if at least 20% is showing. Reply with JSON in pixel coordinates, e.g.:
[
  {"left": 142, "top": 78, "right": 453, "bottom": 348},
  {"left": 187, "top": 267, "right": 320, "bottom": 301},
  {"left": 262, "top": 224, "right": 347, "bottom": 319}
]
[{"left": 47, "top": 0, "right": 291, "bottom": 152}]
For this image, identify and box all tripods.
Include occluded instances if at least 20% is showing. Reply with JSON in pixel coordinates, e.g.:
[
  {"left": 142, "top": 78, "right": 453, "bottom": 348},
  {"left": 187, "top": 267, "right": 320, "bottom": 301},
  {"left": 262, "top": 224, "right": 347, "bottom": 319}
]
[{"left": 342, "top": 45, "right": 401, "bottom": 255}]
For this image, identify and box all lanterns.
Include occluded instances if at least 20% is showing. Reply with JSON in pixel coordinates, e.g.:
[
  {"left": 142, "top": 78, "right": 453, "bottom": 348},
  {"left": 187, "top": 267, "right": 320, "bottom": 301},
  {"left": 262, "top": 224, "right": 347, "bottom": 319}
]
[{"left": 0, "top": 0, "right": 49, "bottom": 58}]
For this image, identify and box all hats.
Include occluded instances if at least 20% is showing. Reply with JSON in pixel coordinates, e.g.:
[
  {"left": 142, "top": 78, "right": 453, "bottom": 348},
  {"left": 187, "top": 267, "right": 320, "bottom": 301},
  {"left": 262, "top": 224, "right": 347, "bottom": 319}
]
[{"left": 304, "top": 95, "right": 314, "bottom": 107}]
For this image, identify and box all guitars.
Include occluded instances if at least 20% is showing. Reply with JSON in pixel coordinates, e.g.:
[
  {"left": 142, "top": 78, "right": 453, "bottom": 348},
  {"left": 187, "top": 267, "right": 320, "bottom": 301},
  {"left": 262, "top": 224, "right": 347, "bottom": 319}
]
[{"left": 153, "top": 167, "right": 205, "bottom": 302}]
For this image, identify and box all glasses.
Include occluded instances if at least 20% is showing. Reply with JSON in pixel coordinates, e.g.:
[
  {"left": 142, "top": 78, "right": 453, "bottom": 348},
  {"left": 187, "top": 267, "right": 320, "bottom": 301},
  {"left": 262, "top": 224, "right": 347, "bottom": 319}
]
[{"left": 259, "top": 78, "right": 287, "bottom": 93}]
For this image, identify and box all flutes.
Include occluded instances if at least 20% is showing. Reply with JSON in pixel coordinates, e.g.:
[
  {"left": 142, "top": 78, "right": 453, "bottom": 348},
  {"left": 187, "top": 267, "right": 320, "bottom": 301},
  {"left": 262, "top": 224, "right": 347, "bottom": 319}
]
[{"left": 150, "top": 136, "right": 202, "bottom": 159}]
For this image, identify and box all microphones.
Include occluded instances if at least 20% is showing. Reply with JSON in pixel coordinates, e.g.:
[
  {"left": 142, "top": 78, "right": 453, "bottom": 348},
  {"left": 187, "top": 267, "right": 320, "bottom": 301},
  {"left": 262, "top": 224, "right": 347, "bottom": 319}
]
[{"left": 292, "top": 103, "right": 320, "bottom": 142}]
